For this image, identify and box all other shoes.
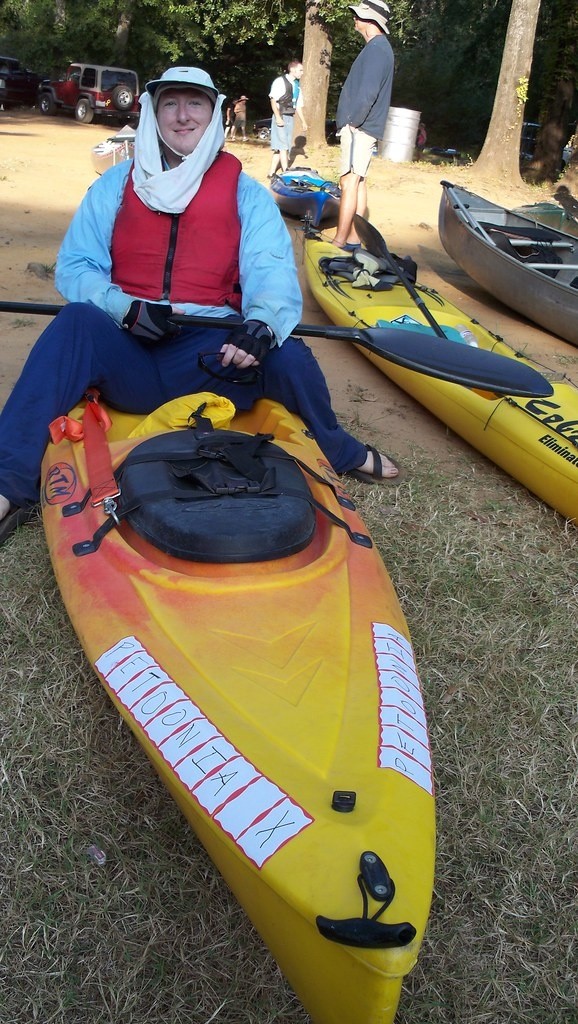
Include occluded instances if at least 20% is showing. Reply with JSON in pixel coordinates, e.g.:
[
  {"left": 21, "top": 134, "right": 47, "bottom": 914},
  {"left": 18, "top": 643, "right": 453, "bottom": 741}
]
[
  {"left": 228, "top": 138, "right": 235, "bottom": 142},
  {"left": 267, "top": 173, "right": 275, "bottom": 178},
  {"left": 242, "top": 138, "right": 248, "bottom": 141}
]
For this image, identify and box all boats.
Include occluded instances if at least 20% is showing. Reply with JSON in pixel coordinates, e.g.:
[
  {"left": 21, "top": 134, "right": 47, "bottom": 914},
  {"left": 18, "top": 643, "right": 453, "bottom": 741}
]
[
  {"left": 41, "top": 381, "right": 438, "bottom": 1022},
  {"left": 437, "top": 178, "right": 578, "bottom": 350},
  {"left": 266, "top": 164, "right": 347, "bottom": 229},
  {"left": 301, "top": 209, "right": 577, "bottom": 530}
]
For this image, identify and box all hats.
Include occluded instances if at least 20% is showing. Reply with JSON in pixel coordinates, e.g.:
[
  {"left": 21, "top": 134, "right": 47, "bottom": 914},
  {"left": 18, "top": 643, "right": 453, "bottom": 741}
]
[
  {"left": 348, "top": 0, "right": 390, "bottom": 35},
  {"left": 236, "top": 95, "right": 249, "bottom": 102},
  {"left": 145, "top": 66, "right": 220, "bottom": 98}
]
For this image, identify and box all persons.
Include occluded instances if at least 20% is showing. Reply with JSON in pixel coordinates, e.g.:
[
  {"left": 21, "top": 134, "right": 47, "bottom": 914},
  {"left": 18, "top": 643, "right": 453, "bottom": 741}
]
[
  {"left": 267, "top": 61, "right": 307, "bottom": 179},
  {"left": 0, "top": 66, "right": 403, "bottom": 544},
  {"left": 224, "top": 98, "right": 239, "bottom": 139},
  {"left": 331, "top": 0, "right": 394, "bottom": 248},
  {"left": 416, "top": 123, "right": 427, "bottom": 152},
  {"left": 230, "top": 96, "right": 249, "bottom": 142}
]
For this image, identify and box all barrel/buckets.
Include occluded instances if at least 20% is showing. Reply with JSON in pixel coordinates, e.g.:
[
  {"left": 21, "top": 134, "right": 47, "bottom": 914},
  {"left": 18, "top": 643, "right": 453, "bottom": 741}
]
[{"left": 377, "top": 105, "right": 423, "bottom": 163}]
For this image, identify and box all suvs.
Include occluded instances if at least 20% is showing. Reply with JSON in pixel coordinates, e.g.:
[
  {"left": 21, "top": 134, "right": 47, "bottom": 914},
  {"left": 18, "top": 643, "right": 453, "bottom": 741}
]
[
  {"left": 36, "top": 63, "right": 142, "bottom": 130},
  {"left": 0, "top": 57, "right": 42, "bottom": 113}
]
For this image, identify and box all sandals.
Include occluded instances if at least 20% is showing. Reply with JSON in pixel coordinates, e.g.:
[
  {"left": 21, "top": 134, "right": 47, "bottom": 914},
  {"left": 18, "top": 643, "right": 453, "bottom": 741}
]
[
  {"left": 347, "top": 444, "right": 407, "bottom": 486},
  {"left": 0, "top": 500, "right": 36, "bottom": 549}
]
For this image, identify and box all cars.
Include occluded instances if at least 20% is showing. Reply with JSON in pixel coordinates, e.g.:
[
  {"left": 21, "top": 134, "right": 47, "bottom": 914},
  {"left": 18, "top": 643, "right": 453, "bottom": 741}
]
[{"left": 253, "top": 113, "right": 337, "bottom": 145}]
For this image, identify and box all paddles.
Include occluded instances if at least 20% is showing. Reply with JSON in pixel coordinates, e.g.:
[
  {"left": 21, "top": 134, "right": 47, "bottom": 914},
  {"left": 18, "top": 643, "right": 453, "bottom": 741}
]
[
  {"left": 353, "top": 214, "right": 448, "bottom": 339},
  {"left": 441, "top": 180, "right": 496, "bottom": 246},
  {"left": 1, "top": 301, "right": 554, "bottom": 399}
]
